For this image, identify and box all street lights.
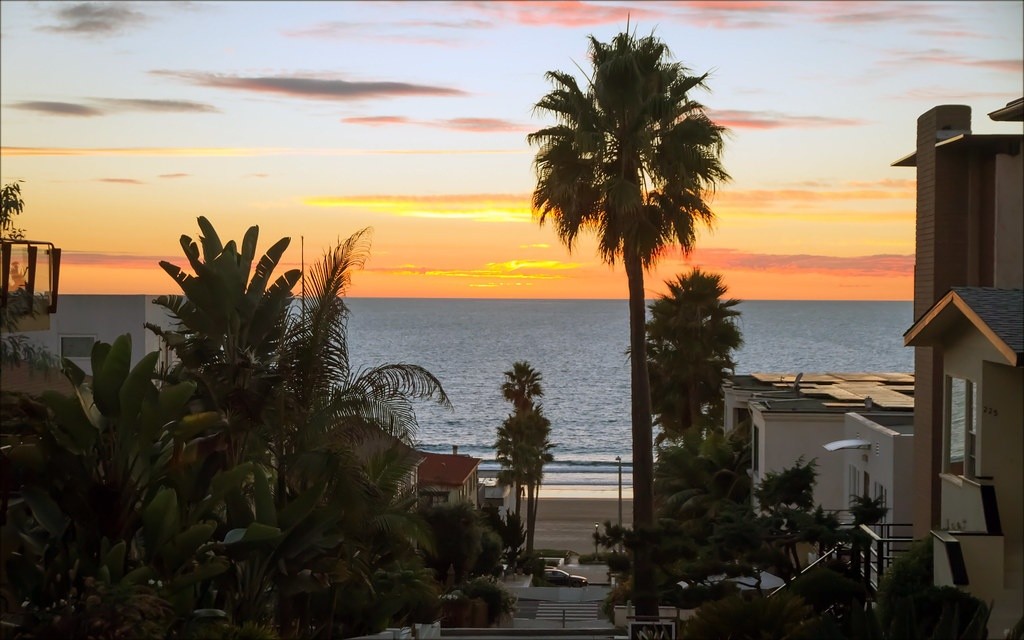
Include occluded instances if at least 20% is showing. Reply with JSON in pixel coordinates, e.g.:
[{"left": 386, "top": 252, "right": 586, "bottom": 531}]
[
  {"left": 615, "top": 455, "right": 622, "bottom": 554},
  {"left": 594, "top": 521, "right": 599, "bottom": 561}
]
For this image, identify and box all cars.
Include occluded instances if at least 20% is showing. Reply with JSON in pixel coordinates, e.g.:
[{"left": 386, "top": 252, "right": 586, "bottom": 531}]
[{"left": 533, "top": 568, "right": 587, "bottom": 587}]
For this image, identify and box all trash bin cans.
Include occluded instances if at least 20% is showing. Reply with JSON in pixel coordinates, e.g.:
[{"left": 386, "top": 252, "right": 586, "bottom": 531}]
[{"left": 609, "top": 571, "right": 620, "bottom": 589}]
